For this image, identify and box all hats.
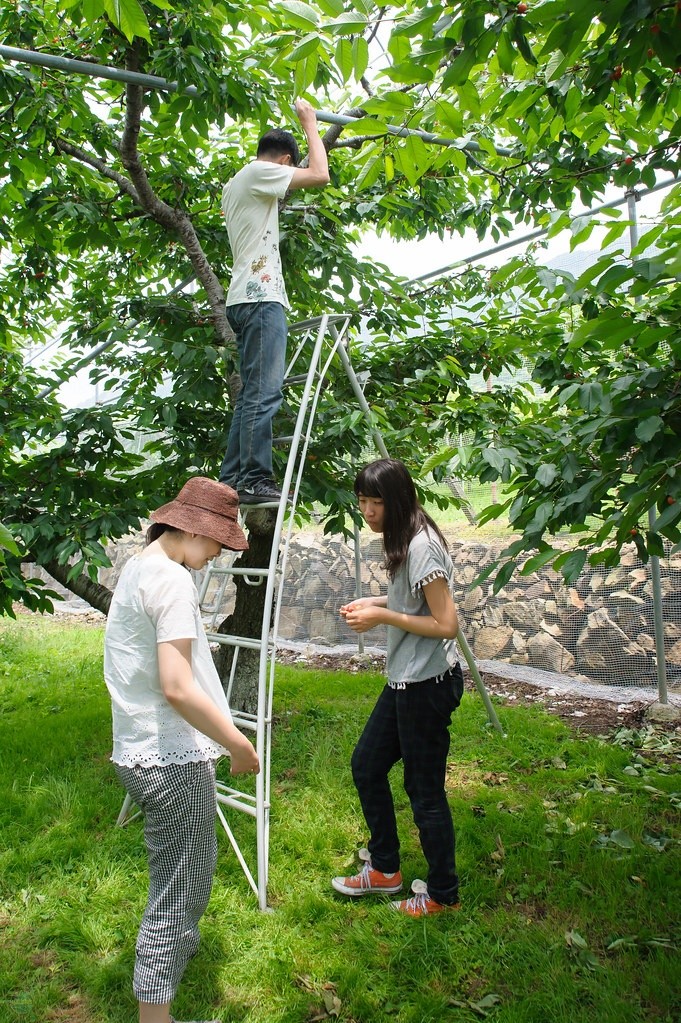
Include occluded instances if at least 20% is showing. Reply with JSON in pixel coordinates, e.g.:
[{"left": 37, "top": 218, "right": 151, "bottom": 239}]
[{"left": 150, "top": 476, "right": 249, "bottom": 551}]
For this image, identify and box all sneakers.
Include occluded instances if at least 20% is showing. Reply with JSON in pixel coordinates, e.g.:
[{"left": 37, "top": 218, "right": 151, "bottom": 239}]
[
  {"left": 332, "top": 848, "right": 403, "bottom": 895},
  {"left": 236, "top": 482, "right": 294, "bottom": 503},
  {"left": 387, "top": 879, "right": 460, "bottom": 917}
]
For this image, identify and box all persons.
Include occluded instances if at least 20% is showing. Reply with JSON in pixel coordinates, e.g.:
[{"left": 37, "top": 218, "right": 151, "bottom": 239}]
[
  {"left": 331, "top": 458, "right": 465, "bottom": 918},
  {"left": 103, "top": 477, "right": 260, "bottom": 1023},
  {"left": 219, "top": 95, "right": 330, "bottom": 508}
]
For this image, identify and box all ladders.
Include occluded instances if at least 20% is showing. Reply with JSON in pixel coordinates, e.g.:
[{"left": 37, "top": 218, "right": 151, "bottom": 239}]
[{"left": 114, "top": 313, "right": 508, "bottom": 909}]
